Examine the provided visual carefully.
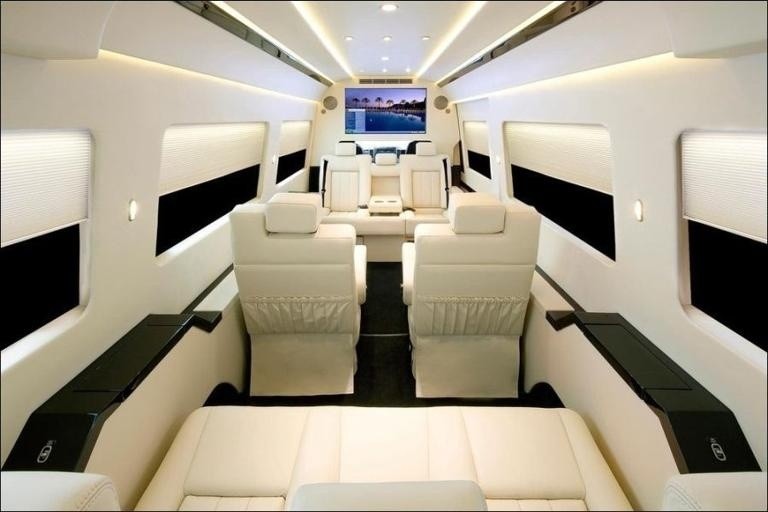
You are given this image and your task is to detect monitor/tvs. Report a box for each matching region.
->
[345,88,427,134]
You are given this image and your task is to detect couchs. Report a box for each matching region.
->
[0,399,765,511]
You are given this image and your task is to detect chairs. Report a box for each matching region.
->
[233,192,368,396]
[402,194,540,399]
[315,142,475,263]
